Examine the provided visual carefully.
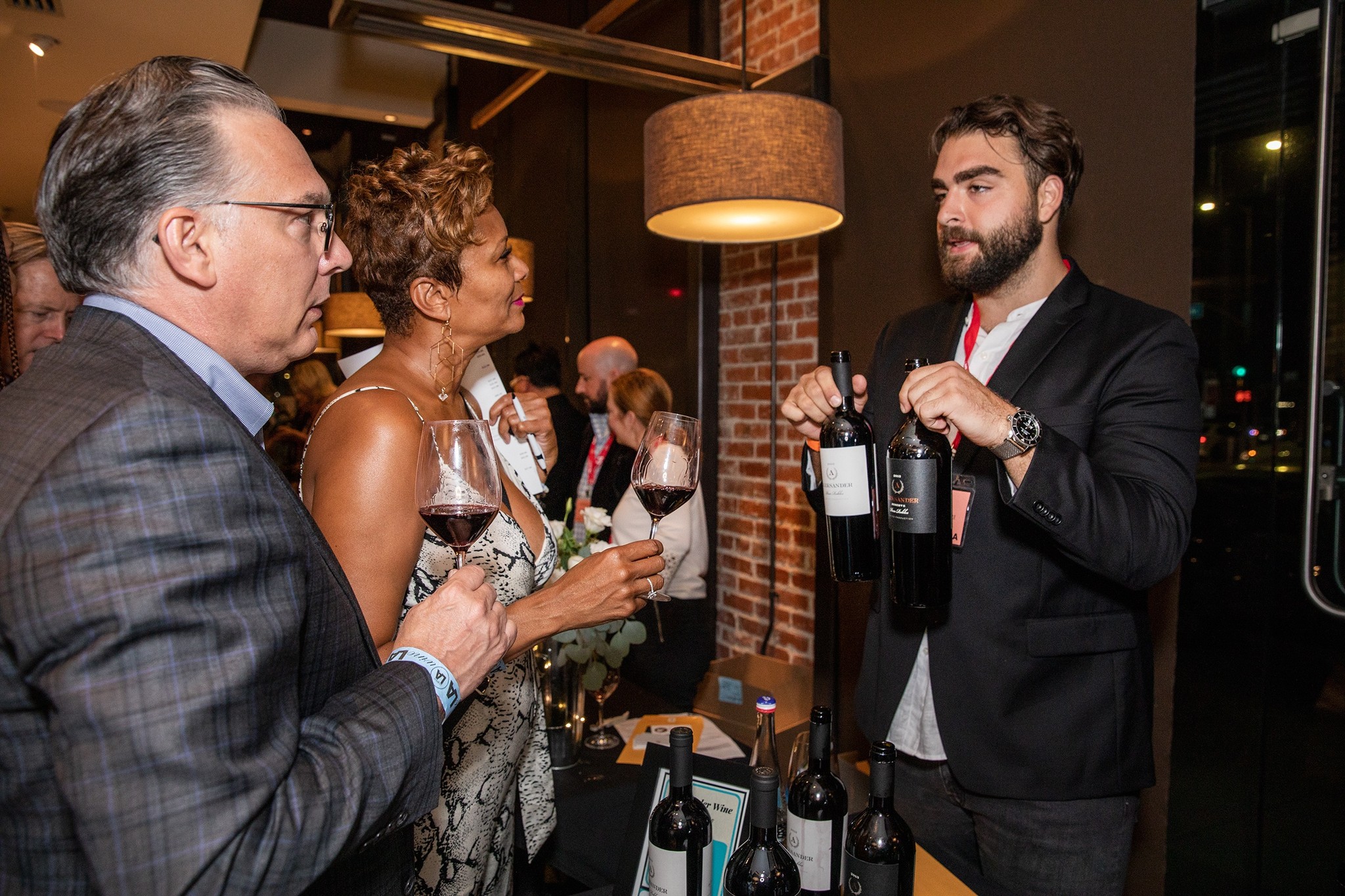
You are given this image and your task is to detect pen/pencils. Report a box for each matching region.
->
[510,392,547,474]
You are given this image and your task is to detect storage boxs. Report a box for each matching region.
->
[691,652,872,814]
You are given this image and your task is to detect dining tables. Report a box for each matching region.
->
[542,671,982,896]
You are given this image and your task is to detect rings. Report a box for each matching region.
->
[647,578,658,600]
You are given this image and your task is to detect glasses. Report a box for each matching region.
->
[153,200,336,252]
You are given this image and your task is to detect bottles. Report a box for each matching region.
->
[818,346,884,584]
[646,688,917,896]
[888,355,953,611]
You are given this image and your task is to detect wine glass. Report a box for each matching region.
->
[582,668,622,750]
[632,411,704,603]
[414,413,503,568]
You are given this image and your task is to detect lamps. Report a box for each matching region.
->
[322,291,387,338]
[311,320,341,354]
[26,33,62,56]
[643,90,846,243]
[504,236,537,303]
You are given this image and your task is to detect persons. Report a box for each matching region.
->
[0,219,77,382]
[1,57,515,896]
[599,367,721,713]
[563,336,640,542]
[779,91,1199,896]
[503,341,593,535]
[295,137,667,893]
[262,358,340,489]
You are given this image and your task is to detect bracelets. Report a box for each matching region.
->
[387,647,461,720]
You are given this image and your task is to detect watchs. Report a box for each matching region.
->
[988,407,1043,460]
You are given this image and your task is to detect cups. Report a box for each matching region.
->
[545,705,582,772]
